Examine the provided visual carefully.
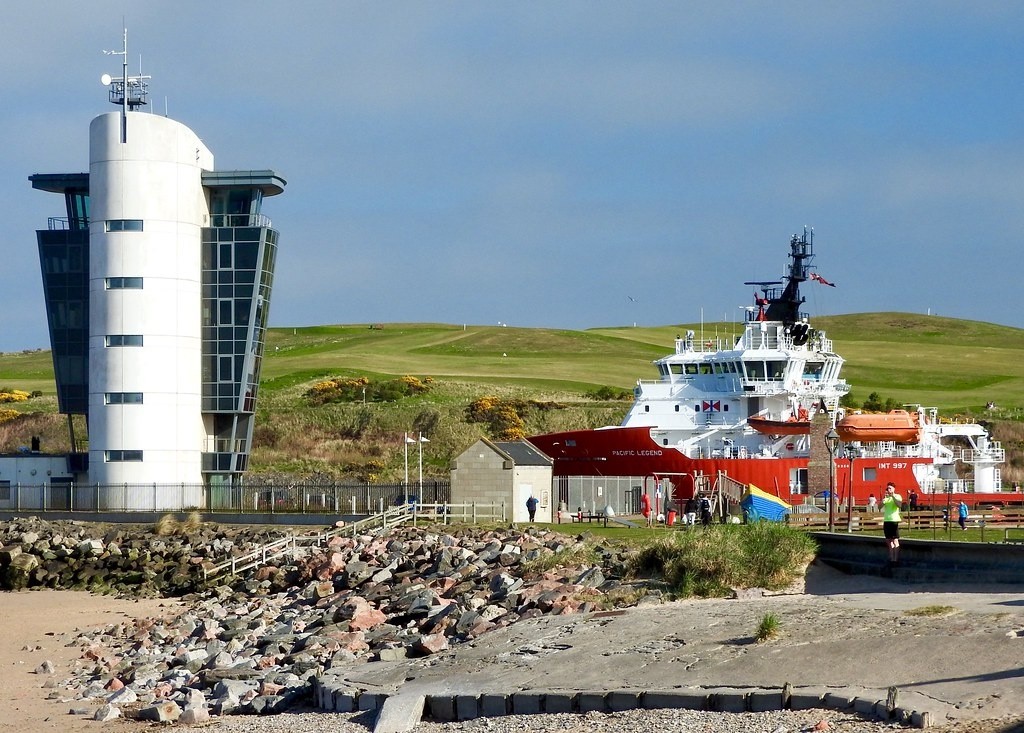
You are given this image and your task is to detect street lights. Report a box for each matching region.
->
[419,437,430,511]
[824,427,840,534]
[844,442,859,533]
[404,437,416,512]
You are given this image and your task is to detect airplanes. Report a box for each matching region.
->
[628,296,639,303]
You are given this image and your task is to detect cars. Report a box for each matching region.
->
[394,494,419,512]
[740,483,793,522]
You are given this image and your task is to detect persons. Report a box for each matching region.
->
[909,489,919,511]
[525,495,539,522]
[951,499,969,530]
[877,481,903,566]
[684,497,699,525]
[699,493,711,525]
[867,494,878,513]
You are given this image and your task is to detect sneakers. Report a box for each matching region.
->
[889,561,899,567]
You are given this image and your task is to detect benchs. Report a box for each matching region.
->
[571,510,609,523]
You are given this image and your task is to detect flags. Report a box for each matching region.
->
[809,272,836,288]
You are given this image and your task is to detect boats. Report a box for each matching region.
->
[526,223,1024,516]
